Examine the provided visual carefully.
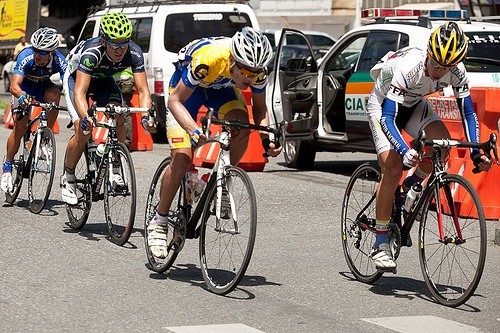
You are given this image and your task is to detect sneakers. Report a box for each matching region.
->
[39,147,52,160]
[1,163,13,193]
[214,185,230,219]
[62,174,78,206]
[368,243,397,269]
[146,216,168,259]
[414,198,425,221]
[113,174,124,192]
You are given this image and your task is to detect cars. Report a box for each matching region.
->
[263,8,500,168]
[0,44,69,92]
[264,29,336,61]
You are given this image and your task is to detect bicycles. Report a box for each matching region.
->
[340,128,499,307]
[6,101,74,214]
[143,107,289,296]
[62,101,159,245]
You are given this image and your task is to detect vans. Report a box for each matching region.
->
[65,0,261,126]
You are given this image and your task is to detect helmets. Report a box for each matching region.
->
[30,26,59,52]
[100,11,133,40]
[230,26,273,70]
[427,22,470,69]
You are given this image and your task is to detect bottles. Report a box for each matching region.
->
[403,181,422,213]
[190,172,211,202]
[185,166,198,205]
[88,140,98,171]
[94,143,104,165]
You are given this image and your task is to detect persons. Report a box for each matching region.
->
[148,25,282,258]
[366,21,491,270]
[13,36,33,60]
[0,26,69,194]
[60,11,156,205]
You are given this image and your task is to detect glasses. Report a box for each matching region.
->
[235,61,264,78]
[428,52,457,71]
[102,36,130,49]
[31,46,49,55]
